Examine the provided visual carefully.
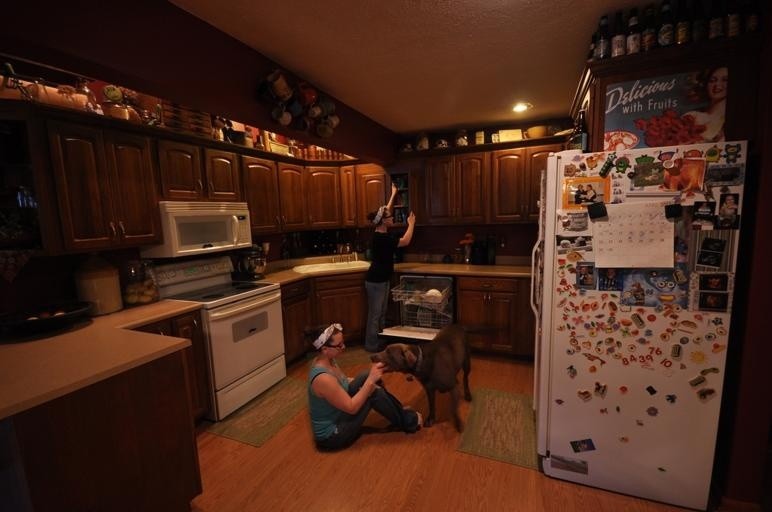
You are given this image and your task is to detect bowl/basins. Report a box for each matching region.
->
[8,299,95,333]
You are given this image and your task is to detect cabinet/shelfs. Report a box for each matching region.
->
[492,137,563,224]
[302,160,342,229]
[241,147,306,235]
[340,160,356,229]
[357,149,426,228]
[157,129,240,202]
[427,143,492,225]
[1,95,164,253]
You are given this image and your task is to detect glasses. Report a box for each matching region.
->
[325,343,343,348]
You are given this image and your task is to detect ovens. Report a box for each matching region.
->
[202,289,287,424]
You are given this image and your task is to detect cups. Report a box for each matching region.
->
[523,125,547,138]
[264,69,342,137]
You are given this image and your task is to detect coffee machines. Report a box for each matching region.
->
[237,242,267,281]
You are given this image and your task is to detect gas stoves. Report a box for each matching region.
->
[163,279,278,309]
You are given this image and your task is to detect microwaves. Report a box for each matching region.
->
[139,199,253,260]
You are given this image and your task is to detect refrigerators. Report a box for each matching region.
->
[530,139,749,510]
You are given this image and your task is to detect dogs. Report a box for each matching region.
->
[369,325,473,433]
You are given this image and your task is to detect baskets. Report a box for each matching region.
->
[391,280,451,304]
[400,309,453,329]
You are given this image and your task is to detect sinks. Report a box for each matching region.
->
[293,259,371,275]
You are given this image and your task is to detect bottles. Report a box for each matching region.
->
[588,0,764,60]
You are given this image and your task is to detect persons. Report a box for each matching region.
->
[584,184,596,201]
[721,195,737,217]
[599,269,618,290]
[306,322,423,453]
[580,267,588,282]
[575,184,585,202]
[683,68,727,141]
[353,181,417,354]
